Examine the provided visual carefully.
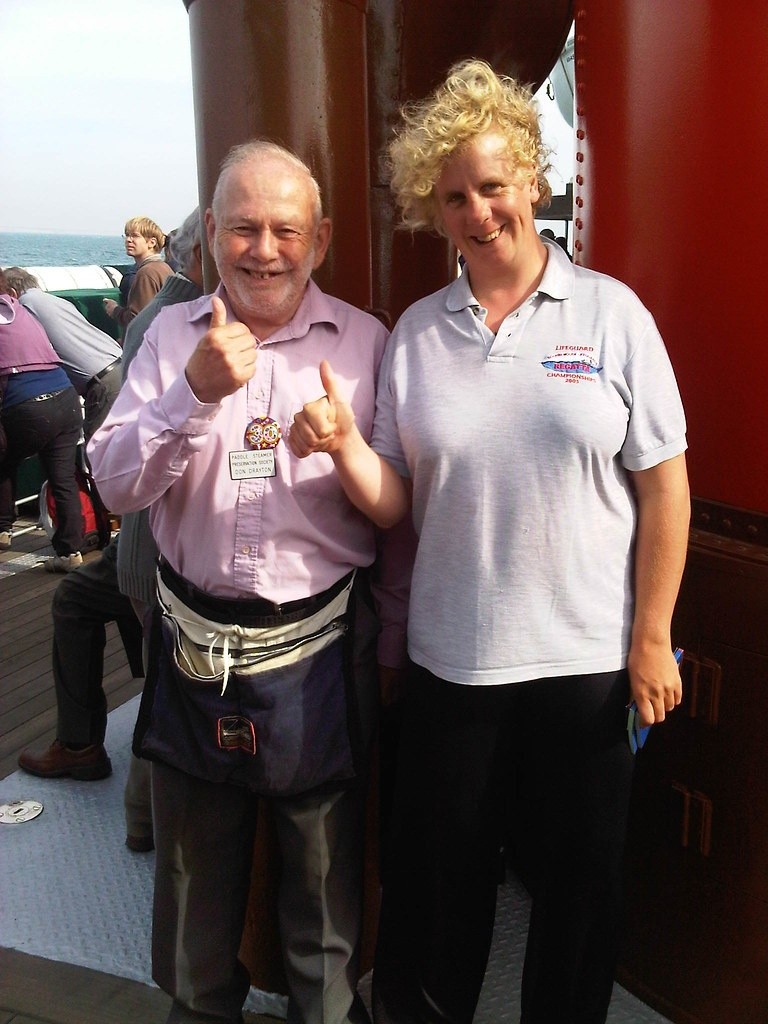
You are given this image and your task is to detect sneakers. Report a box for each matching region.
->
[45,551,84,572]
[0,532,12,550]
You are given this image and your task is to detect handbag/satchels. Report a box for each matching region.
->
[131,569,357,796]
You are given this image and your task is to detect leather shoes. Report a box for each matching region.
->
[18,739,113,781]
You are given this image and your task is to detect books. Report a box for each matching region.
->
[626,649,684,754]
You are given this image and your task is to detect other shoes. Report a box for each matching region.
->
[126,834,154,852]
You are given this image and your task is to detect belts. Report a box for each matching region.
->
[161,558,354,618]
[87,358,122,388]
[33,390,64,401]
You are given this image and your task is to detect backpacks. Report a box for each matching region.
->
[39,462,111,555]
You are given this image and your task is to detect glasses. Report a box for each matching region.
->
[122,233,143,239]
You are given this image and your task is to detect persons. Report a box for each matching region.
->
[291,64,692,1024]
[117,207,205,852]
[0,265,123,575]
[103,216,182,346]
[17,537,126,780]
[86,142,390,1024]
